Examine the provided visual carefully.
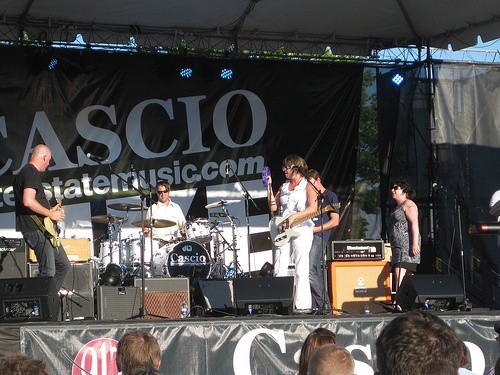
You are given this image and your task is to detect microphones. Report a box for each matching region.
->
[131,164,134,180]
[86,153,107,161]
[291,164,303,170]
[225,160,229,178]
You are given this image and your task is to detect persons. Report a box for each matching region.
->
[299,328,337,375]
[144,180,186,247]
[267,155,317,312]
[13,144,70,293]
[494,322,500,375]
[388,180,421,286]
[115,330,161,375]
[307,170,339,309]
[126,365,160,375]
[308,343,355,375]
[375,310,464,375]
[0,354,48,375]
[489,190,500,245]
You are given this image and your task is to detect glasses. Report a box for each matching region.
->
[282,166,292,171]
[158,190,168,195]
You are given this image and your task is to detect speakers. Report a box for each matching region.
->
[96,285,141,321]
[394,273,464,311]
[190,278,234,317]
[327,260,391,314]
[28,263,94,320]
[0,238,29,278]
[135,277,190,318]
[0,276,61,323]
[231,276,297,314]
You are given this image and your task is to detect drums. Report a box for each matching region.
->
[101,239,129,269]
[152,241,212,279]
[185,217,212,246]
[125,238,160,266]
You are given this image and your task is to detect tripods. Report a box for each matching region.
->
[96,160,172,321]
[301,170,358,315]
[204,206,248,279]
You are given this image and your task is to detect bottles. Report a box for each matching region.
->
[227,268,235,278]
[181,299,187,318]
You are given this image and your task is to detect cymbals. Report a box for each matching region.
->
[130,219,177,228]
[205,199,242,209]
[262,166,271,187]
[107,203,148,211]
[90,216,128,224]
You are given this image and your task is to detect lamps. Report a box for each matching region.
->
[389,66,407,88]
[179,56,194,78]
[219,61,238,82]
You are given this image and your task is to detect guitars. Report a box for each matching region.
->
[44,199,62,247]
[268,201,340,247]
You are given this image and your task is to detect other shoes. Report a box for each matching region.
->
[292,309,313,315]
[383,304,403,312]
